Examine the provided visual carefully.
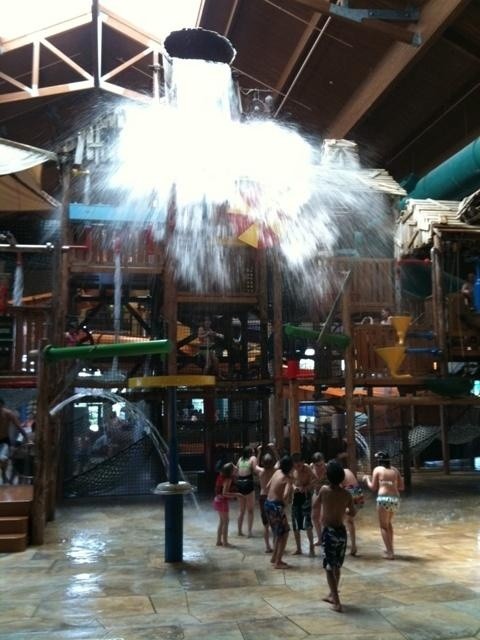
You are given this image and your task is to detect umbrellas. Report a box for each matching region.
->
[227,187,285,251]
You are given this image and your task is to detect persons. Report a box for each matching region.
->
[213,441,366,570]
[198,316,228,382]
[460,272,476,312]
[361,451,405,561]
[380,306,395,325]
[0,397,36,485]
[91,411,123,455]
[308,458,358,614]
